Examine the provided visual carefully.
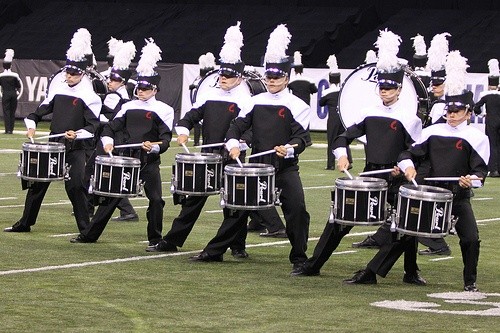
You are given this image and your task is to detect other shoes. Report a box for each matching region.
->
[4,226,12,232]
[247,224,267,232]
[326,166,335,170]
[341,164,353,173]
[259,228,287,238]
[489,170,499,177]
[111,214,139,222]
[149,241,158,246]
[70,236,84,243]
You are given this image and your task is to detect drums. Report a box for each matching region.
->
[221,163,282,210]
[390,185,459,238]
[192,70,267,125]
[329,177,392,225]
[88,155,146,198]
[17,142,72,182]
[170,153,222,196]
[337,62,428,144]
[46,66,108,98]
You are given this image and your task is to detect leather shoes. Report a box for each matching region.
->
[403,273,427,285]
[189,250,224,263]
[232,249,249,258]
[351,236,379,249]
[343,270,378,286]
[292,260,306,270]
[145,240,178,252]
[419,245,451,256]
[464,283,478,293]
[289,261,320,277]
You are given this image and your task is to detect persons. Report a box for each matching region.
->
[247,208,287,238]
[189,67,214,146]
[71,73,174,246]
[290,70,427,286]
[287,64,317,145]
[4,59,103,230]
[0,61,23,133]
[343,91,490,292]
[189,60,311,270]
[474,74,500,177]
[85,54,137,103]
[319,72,353,171]
[145,62,249,257]
[71,69,140,221]
[353,57,451,255]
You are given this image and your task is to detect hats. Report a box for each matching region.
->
[65,58,88,74]
[443,89,474,111]
[488,75,499,86]
[290,63,304,74]
[218,61,245,76]
[328,72,341,84]
[109,68,132,83]
[430,67,446,85]
[378,69,404,89]
[265,60,291,79]
[200,67,214,76]
[2,62,12,69]
[412,53,428,67]
[135,74,162,91]
[6,130,12,134]
